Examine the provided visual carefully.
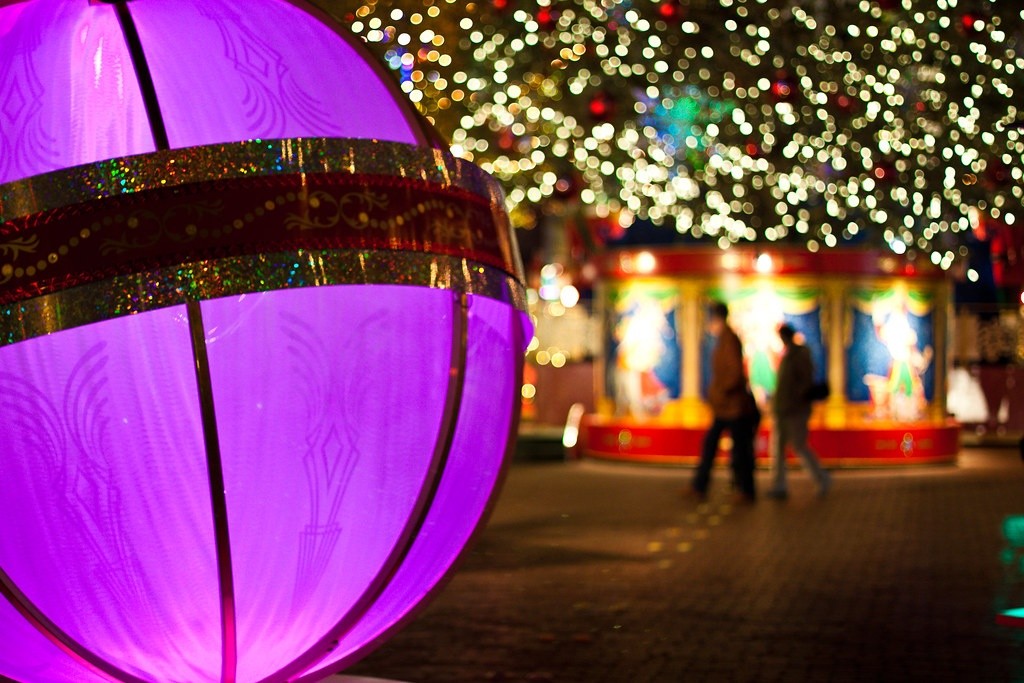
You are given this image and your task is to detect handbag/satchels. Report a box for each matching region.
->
[809,379,830,401]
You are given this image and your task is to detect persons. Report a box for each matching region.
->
[766,322,832,500]
[680,302,756,503]
